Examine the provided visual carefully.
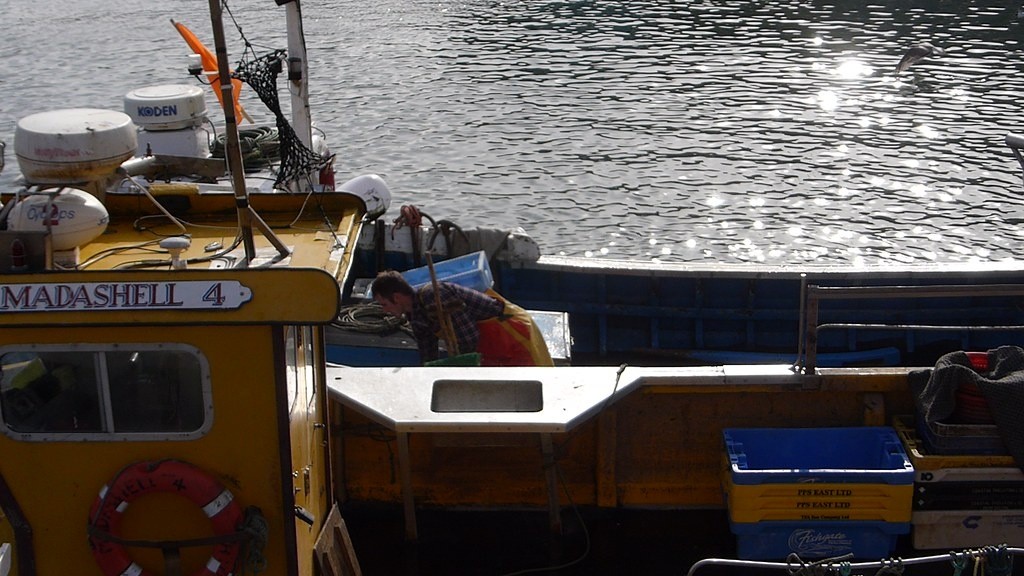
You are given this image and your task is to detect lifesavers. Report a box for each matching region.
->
[90,461,242,576]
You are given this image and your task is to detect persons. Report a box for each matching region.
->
[372,271,555,367]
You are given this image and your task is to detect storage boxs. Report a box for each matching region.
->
[715,408,1024,563]
[404,250,495,292]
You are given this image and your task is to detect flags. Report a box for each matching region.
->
[173,22,243,125]
